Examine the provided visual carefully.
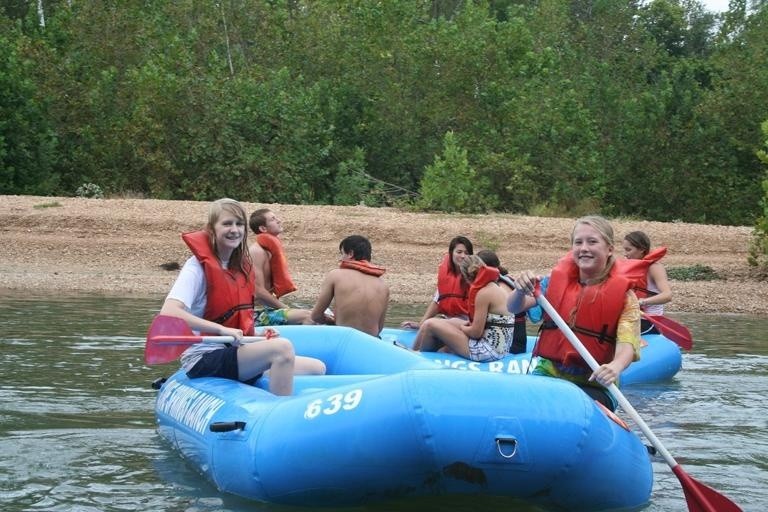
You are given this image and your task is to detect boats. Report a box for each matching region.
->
[379,324,681,385]
[154,325,654,512]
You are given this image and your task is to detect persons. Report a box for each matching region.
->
[400,236,473,351]
[310,234,389,338]
[247,209,319,328]
[160,197,327,395]
[622,231,673,336]
[414,250,516,363]
[506,215,642,414]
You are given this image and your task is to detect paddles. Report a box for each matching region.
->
[144,316,274,366]
[532,278,742,512]
[640,310,693,350]
